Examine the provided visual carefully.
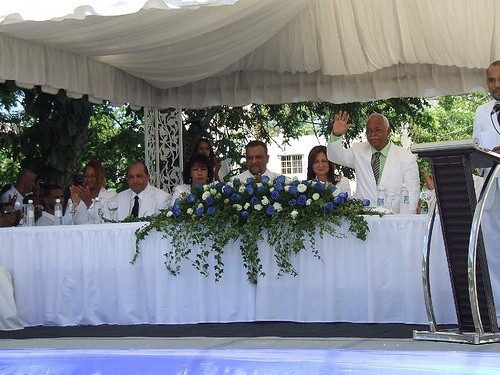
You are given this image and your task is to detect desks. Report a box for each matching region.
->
[0,215,457,330]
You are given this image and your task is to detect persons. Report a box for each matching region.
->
[0,139,221,227]
[327,111,420,213]
[471,60,500,178]
[229,140,282,184]
[420,167,483,214]
[307,145,351,197]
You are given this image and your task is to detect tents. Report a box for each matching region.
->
[0,0,500,196]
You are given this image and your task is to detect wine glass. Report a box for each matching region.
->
[107,201,118,223]
[66,203,78,225]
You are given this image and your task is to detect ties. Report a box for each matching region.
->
[372,152,381,182]
[129,196,139,218]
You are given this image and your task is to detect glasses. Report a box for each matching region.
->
[3,210,15,215]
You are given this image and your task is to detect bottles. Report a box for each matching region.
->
[54,199,63,225]
[377,186,386,207]
[94,197,102,224]
[26,199,35,226]
[399,184,410,214]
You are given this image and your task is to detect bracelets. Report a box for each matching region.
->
[333,132,343,137]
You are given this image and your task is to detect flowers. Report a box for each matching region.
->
[98,175,393,284]
